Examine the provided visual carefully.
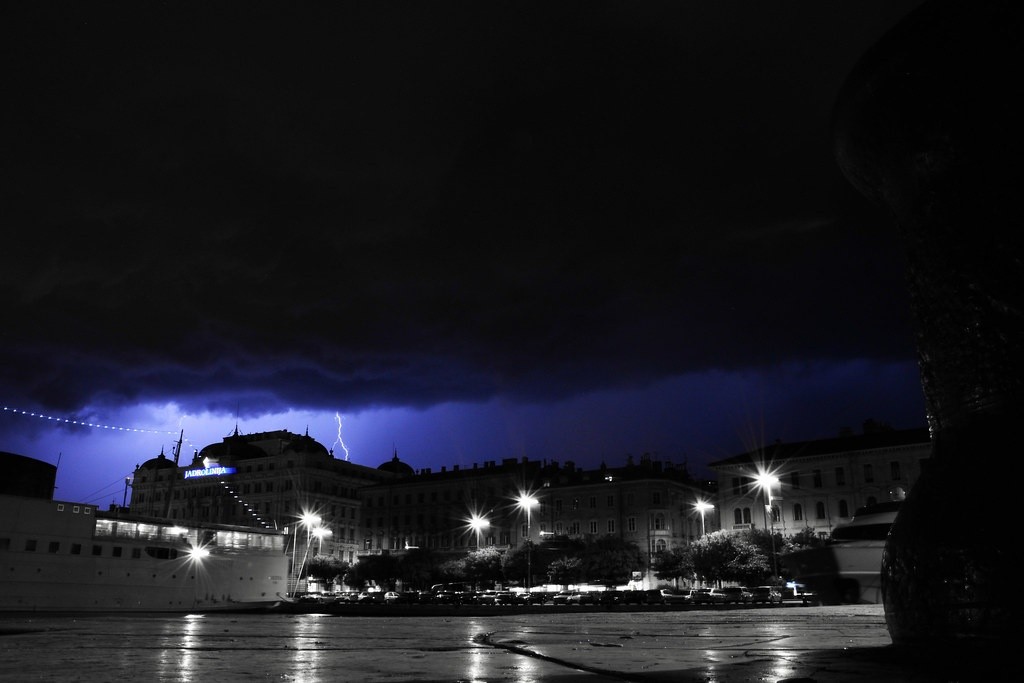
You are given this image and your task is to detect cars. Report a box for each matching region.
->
[286,592,309,602]
[320,591,339,604]
[478,586,823,607]
[350,593,358,602]
[335,591,356,604]
[301,591,322,602]
[384,591,409,604]
[358,591,375,602]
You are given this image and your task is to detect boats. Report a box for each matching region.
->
[0,492,291,618]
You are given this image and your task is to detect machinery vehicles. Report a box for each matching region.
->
[431,581,477,605]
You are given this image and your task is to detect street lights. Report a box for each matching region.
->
[123,477,130,507]
[695,501,707,538]
[316,528,326,558]
[759,472,781,586]
[521,494,535,589]
[471,516,485,551]
[301,512,315,558]
